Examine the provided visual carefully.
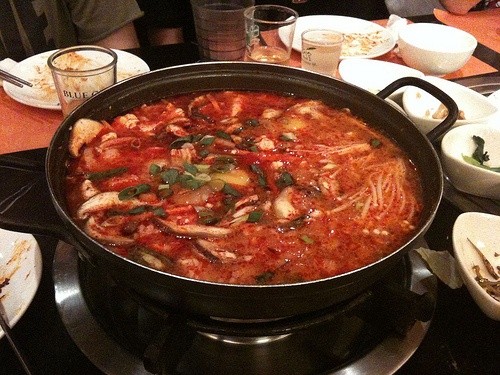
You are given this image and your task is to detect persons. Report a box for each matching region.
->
[0,0,499,66]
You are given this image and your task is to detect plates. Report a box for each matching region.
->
[0,229,42,337]
[2,49,150,111]
[278,14,396,60]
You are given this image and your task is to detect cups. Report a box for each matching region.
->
[48,44,118,120]
[301,30,344,78]
[244,5,298,66]
[190,0,255,62]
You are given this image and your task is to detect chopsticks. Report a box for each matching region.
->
[0,69,33,87]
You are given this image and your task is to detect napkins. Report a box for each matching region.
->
[0,86,64,155]
[433,7,500,54]
[260,18,498,80]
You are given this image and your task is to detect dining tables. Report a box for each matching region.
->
[0,13,500,375]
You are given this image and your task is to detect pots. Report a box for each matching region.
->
[43,61,458,317]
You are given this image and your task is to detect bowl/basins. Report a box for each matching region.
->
[453,212,500,323]
[399,22,477,75]
[401,77,498,139]
[440,124,500,200]
[339,59,425,100]
